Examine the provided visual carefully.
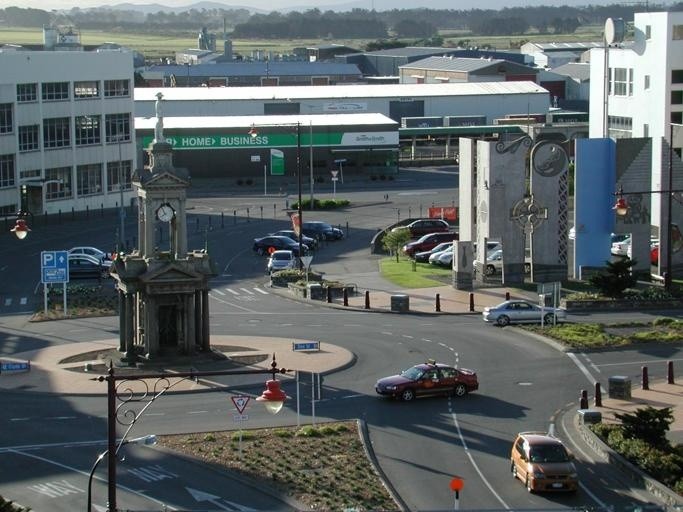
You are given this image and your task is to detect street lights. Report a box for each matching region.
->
[87,434,157,512]
[0,210,34,241]
[611,184,683,216]
[87,352,297,512]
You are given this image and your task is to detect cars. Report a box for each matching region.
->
[252,221,344,276]
[511,431,579,494]
[568,226,659,267]
[482,300,567,327]
[374,358,479,401]
[67,246,114,279]
[391,218,531,277]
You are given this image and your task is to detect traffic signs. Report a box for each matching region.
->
[293,340,321,353]
[41,250,69,284]
[0,362,31,373]
